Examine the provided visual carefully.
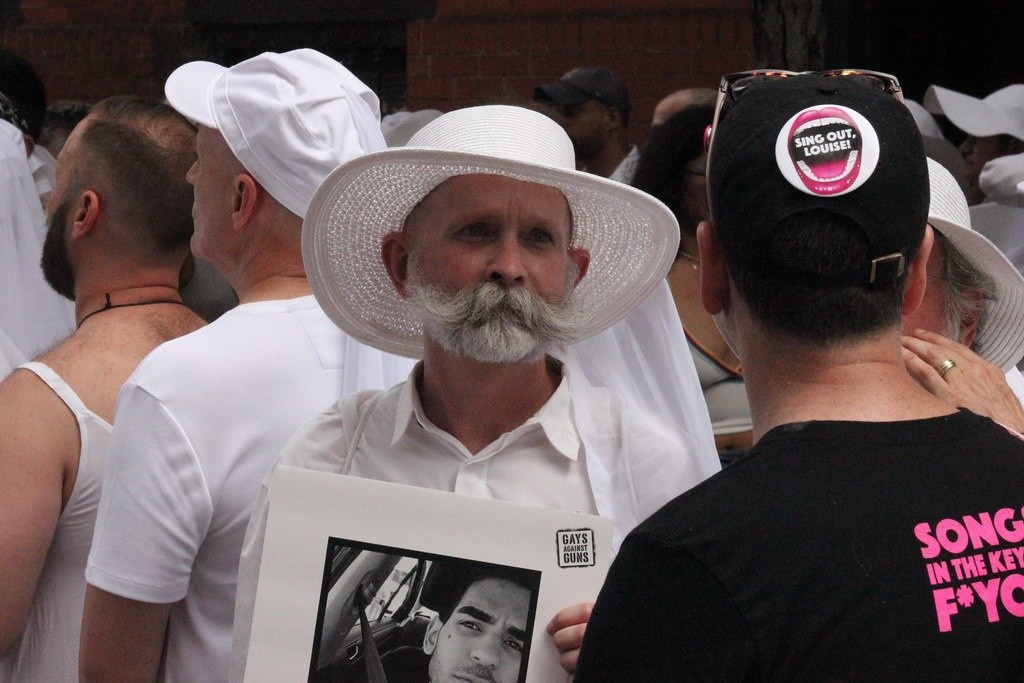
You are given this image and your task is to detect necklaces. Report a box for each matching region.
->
[77,293,185,330]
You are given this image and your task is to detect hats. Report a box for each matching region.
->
[711,74,929,284]
[927,156,1024,375]
[978,152,1024,208]
[301,104,681,358]
[903,99,969,193]
[380,110,443,148]
[533,65,632,114]
[923,84,1024,142]
[163,47,388,219]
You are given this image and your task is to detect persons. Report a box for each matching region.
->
[534,65,640,186]
[0,49,95,378]
[0,94,210,683]
[78,48,420,683]
[571,67,1024,683]
[227,105,683,683]
[423,562,532,683]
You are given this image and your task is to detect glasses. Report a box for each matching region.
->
[546,103,605,118]
[705,68,905,246]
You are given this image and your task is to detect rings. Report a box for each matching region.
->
[938,359,955,378]
